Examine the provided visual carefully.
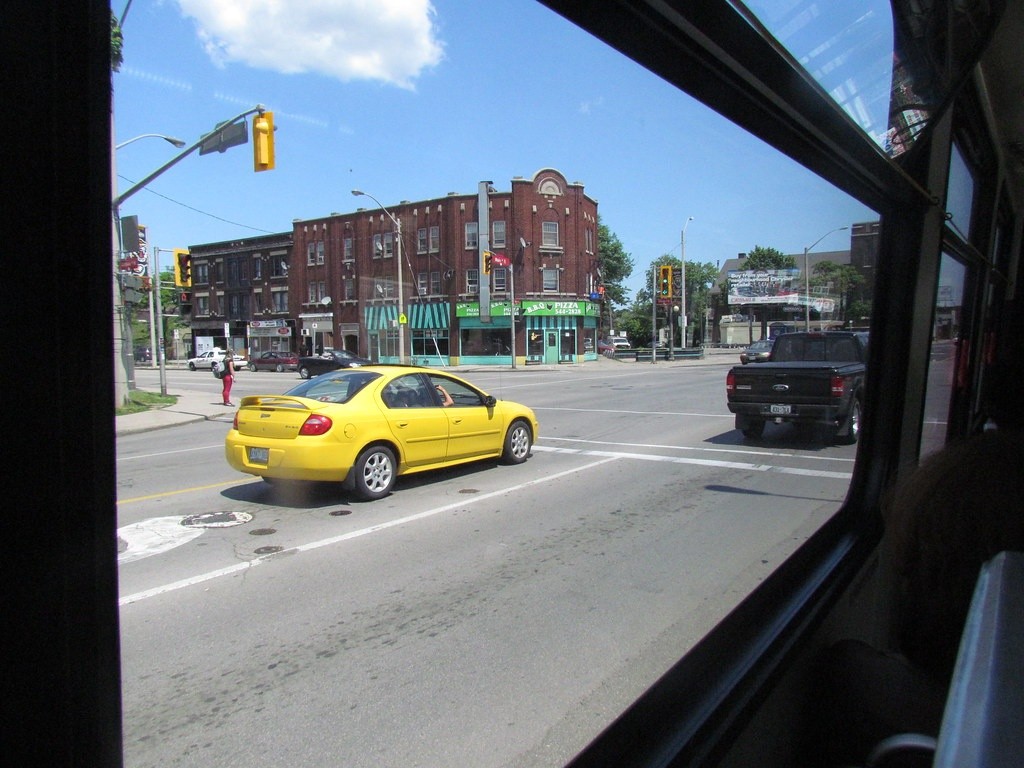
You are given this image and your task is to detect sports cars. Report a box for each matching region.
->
[299,348,374,379]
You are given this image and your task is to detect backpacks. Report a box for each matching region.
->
[213,358,232,379]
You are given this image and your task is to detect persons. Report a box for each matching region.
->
[416,380,454,408]
[221,349,237,406]
[299,341,309,357]
[878,424,1024,670]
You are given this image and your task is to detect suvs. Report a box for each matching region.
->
[610,337,631,349]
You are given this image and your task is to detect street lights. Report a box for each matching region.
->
[115,133,186,151]
[351,190,405,366]
[483,251,492,274]
[681,216,694,349]
[804,226,849,331]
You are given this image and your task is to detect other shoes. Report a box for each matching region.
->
[226,402,234,407]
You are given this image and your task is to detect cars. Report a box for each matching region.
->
[598,340,615,354]
[187,347,248,373]
[740,340,775,366]
[225,364,540,500]
[247,351,299,373]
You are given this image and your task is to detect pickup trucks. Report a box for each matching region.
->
[724,331,869,446]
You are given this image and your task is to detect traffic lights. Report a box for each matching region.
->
[174,249,193,287]
[179,292,193,317]
[660,266,672,299]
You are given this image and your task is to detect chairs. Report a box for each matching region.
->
[386,386,422,407]
